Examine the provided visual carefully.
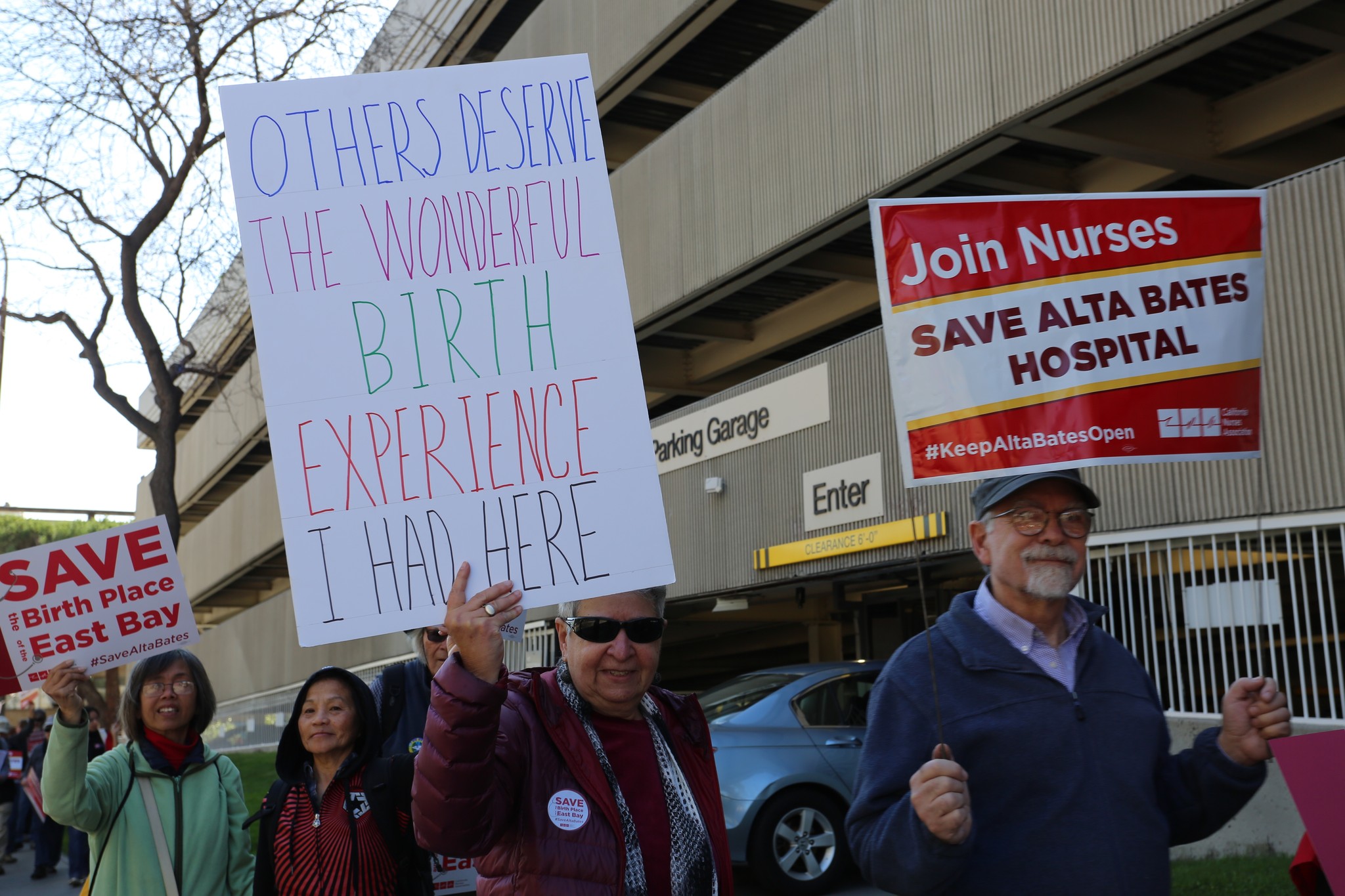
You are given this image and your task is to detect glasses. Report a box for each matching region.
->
[423,626,448,643]
[141,680,195,698]
[978,505,1097,539]
[562,616,667,643]
[45,727,52,732]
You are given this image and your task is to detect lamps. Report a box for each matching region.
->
[704,477,723,494]
[795,587,805,608]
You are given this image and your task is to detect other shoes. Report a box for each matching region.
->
[0,866,6,876]
[31,868,58,880]
[4,854,16,864]
[68,876,86,887]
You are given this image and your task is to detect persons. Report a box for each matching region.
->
[845,470,1294,896]
[0,560,733,896]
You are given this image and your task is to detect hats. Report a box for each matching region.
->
[970,467,1102,522]
[43,715,55,729]
[0,717,10,733]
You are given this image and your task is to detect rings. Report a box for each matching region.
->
[482,604,495,617]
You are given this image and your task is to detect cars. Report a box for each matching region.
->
[694,656,887,896]
